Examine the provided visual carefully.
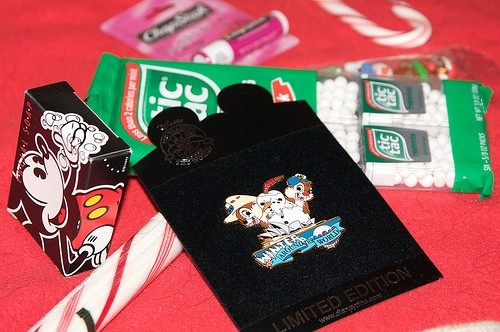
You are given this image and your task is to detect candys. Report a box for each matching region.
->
[316,0,453,189]
